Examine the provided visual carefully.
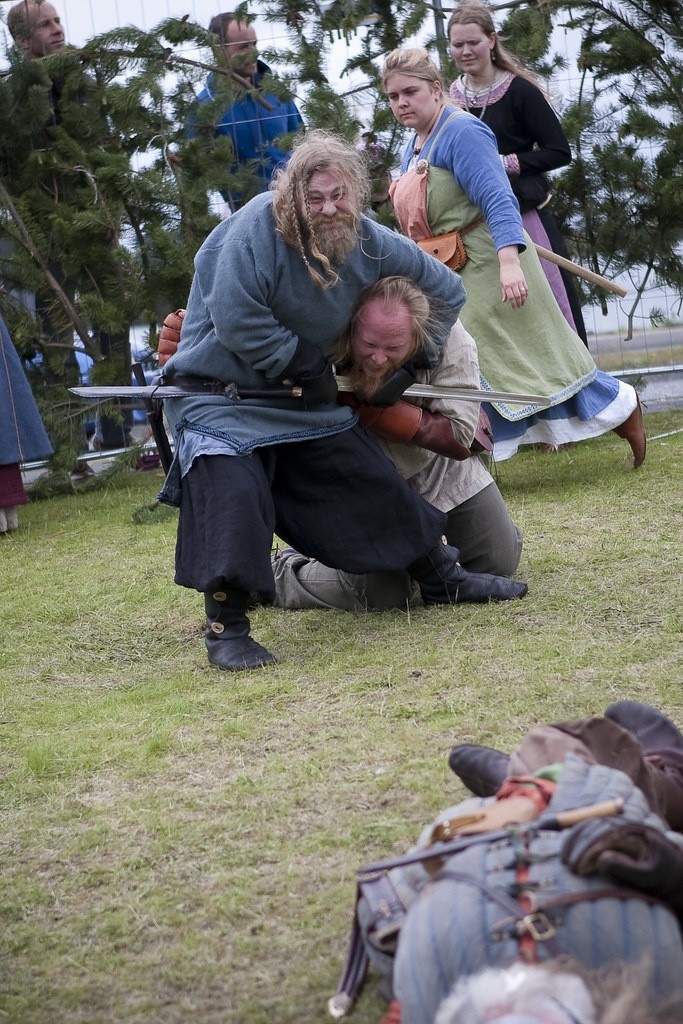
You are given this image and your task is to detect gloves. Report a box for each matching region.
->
[561,814,683,914]
[157,307,190,367]
[301,360,338,409]
[365,366,415,407]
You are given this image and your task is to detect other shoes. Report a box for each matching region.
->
[605,701,683,778]
[90,434,160,470]
[0,506,19,536]
[611,392,646,470]
[449,744,511,798]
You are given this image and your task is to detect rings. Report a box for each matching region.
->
[520,288,525,291]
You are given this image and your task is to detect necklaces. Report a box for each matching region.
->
[465,72,495,121]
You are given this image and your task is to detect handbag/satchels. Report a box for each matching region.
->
[418,230,467,272]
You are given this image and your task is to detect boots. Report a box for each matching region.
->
[407,533,528,607]
[204,577,277,672]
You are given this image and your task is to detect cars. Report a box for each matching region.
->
[25,326,165,434]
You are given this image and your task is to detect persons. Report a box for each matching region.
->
[328,702,683,1023]
[381,48,646,468]
[445,3,588,355]
[179,13,307,215]
[7,0,159,482]
[358,133,394,186]
[158,278,522,612]
[156,134,530,669]
[0,319,54,533]
